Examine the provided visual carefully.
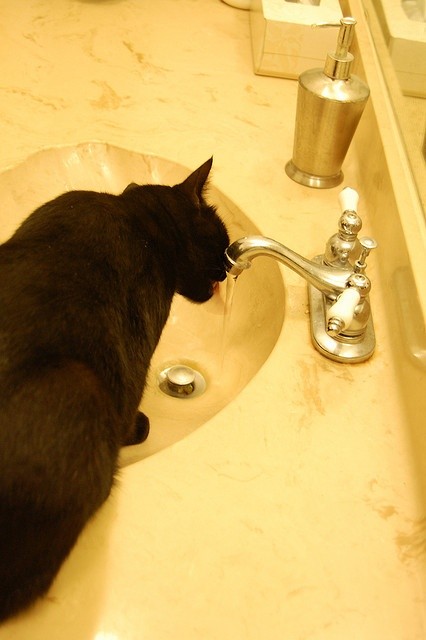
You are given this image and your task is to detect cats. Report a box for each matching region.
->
[1,154,230,623]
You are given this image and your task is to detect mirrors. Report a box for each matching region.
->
[357,0,426,220]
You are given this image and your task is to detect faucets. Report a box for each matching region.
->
[224,202,376,365]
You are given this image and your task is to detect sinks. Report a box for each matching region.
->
[0,136,288,483]
